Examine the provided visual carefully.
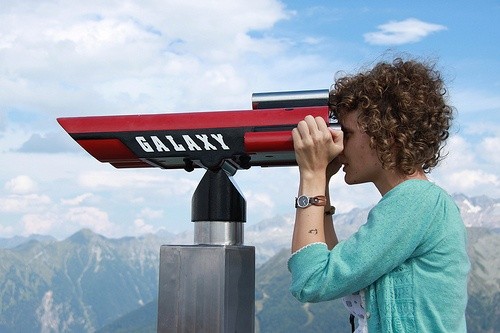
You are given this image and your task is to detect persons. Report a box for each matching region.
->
[287,59,472,333]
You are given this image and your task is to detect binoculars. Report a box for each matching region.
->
[56,89,345,172]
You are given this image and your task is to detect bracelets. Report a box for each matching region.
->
[325,206,335,215]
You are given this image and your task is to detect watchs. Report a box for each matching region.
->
[294,195,328,209]
